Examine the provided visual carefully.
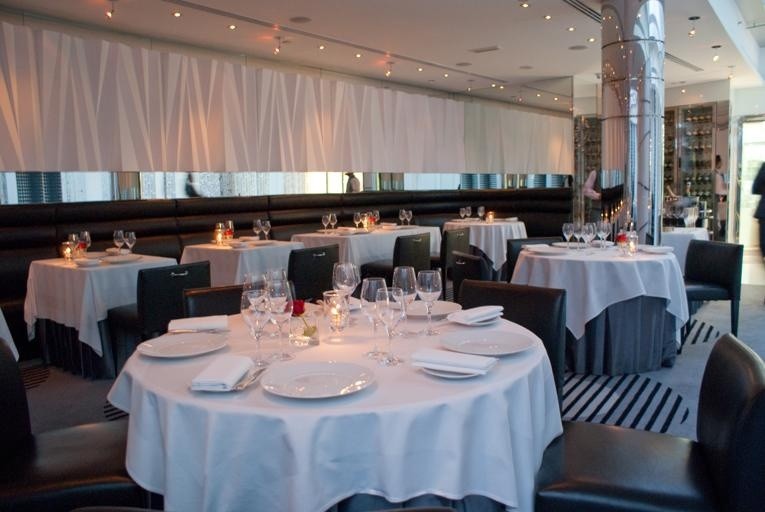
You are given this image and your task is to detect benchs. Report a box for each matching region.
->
[0,187,579,383]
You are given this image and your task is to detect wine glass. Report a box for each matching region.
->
[60,228,136,263]
[563,219,638,256]
[316,208,413,235]
[458,204,495,224]
[237,259,444,371]
[213,218,272,249]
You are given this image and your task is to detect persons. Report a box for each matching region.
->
[583,169,603,222]
[752,161,765,260]
[714,155,727,202]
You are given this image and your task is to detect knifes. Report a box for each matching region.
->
[234,366,266,391]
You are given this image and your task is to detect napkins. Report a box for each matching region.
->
[402,347,497,376]
[189,353,256,393]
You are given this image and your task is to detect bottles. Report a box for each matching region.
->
[575,113,713,233]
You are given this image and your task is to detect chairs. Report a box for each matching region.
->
[677,240,744,345]
[535,332,765,511]
[0,338,163,510]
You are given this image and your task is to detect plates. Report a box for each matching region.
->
[259,357,377,399]
[403,300,462,320]
[447,311,501,326]
[436,328,534,357]
[133,331,227,359]
[421,365,477,380]
[525,240,674,256]
[70,255,141,267]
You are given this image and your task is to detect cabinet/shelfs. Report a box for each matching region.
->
[574,104,721,234]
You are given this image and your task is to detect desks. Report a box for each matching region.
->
[107,301,565,511]
[510,245,688,376]
